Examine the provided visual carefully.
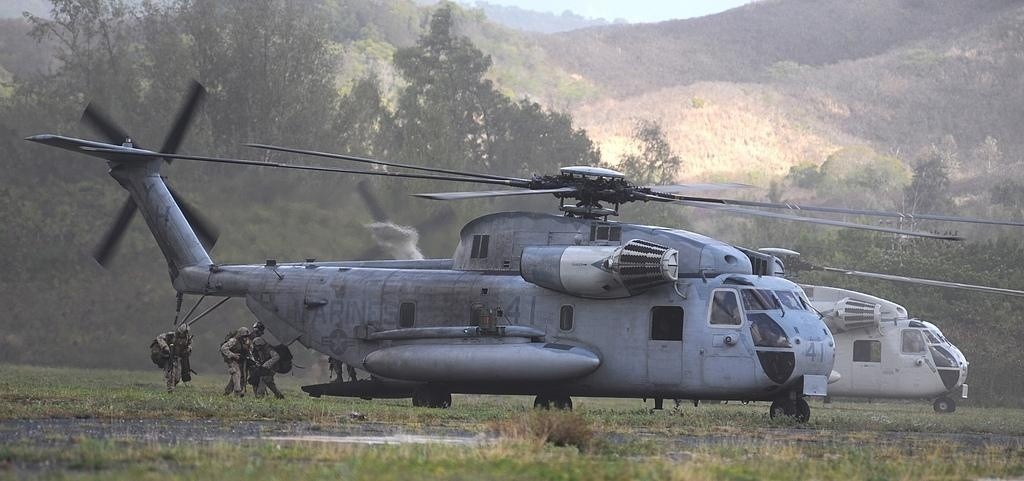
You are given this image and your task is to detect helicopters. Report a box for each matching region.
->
[26,80,1024,427]
[356,173,1024,415]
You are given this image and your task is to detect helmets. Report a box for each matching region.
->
[253,321,264,331]
[238,327,250,337]
[252,336,267,346]
[177,322,190,333]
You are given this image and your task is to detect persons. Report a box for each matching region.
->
[156,323,193,394]
[219,321,284,401]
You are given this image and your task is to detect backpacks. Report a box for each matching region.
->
[269,343,293,375]
[149,338,171,368]
